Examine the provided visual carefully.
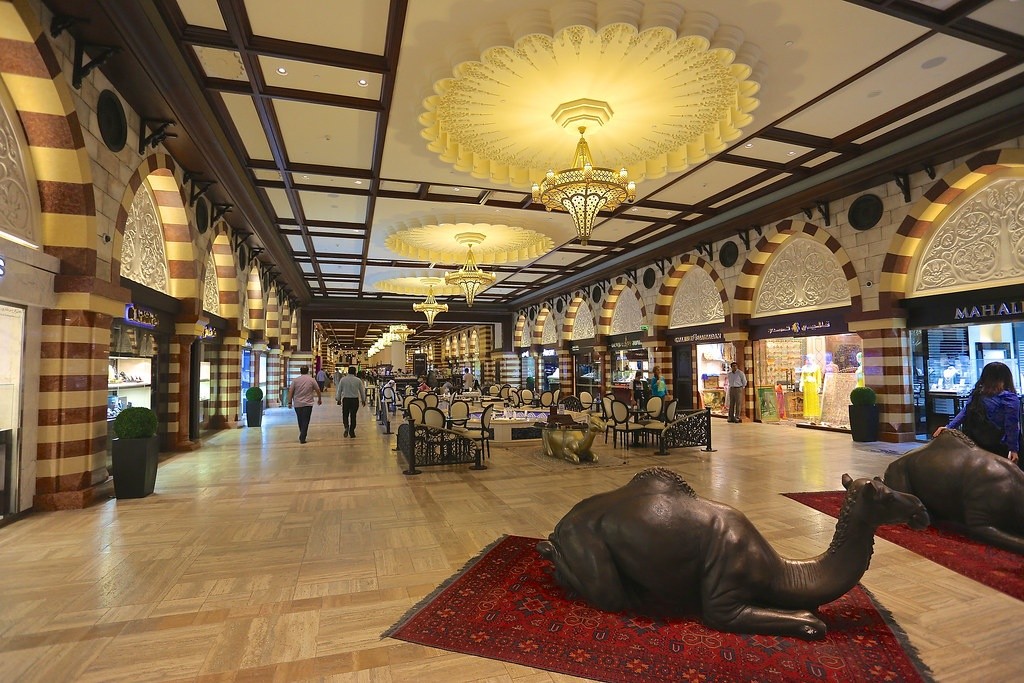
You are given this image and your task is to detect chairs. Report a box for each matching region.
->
[364,379,678,460]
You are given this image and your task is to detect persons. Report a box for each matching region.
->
[855,352,863,387]
[651,366,668,414]
[798,354,821,421]
[932,361,1020,464]
[728,362,747,423]
[428,364,438,387]
[629,371,643,411]
[397,368,404,373]
[317,368,327,392]
[943,366,957,380]
[441,378,453,394]
[821,353,839,374]
[333,368,341,386]
[288,365,322,443]
[335,367,366,437]
[368,369,380,383]
[463,368,481,391]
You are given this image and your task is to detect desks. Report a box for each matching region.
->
[703,389,725,410]
[628,410,656,447]
[524,398,541,408]
[445,418,471,463]
[583,402,601,412]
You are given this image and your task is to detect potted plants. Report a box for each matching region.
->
[246,387,265,426]
[111,407,160,499]
[849,388,877,442]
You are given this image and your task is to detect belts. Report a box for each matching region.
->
[731,387,741,388]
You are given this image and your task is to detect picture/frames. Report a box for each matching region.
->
[755,385,781,422]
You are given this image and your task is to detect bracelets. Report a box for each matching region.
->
[288,403,292,404]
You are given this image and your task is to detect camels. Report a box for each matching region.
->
[540,414,608,464]
[885,428,1024,555]
[534,466,932,644]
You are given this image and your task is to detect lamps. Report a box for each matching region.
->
[413,285,448,329]
[368,322,416,358]
[531,125,636,245]
[444,244,496,308]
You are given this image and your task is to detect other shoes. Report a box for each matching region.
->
[349,430,356,438]
[344,428,349,438]
[735,420,740,423]
[728,420,734,423]
[299,436,307,444]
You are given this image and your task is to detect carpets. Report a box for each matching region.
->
[379,532,939,683]
[779,489,1024,603]
